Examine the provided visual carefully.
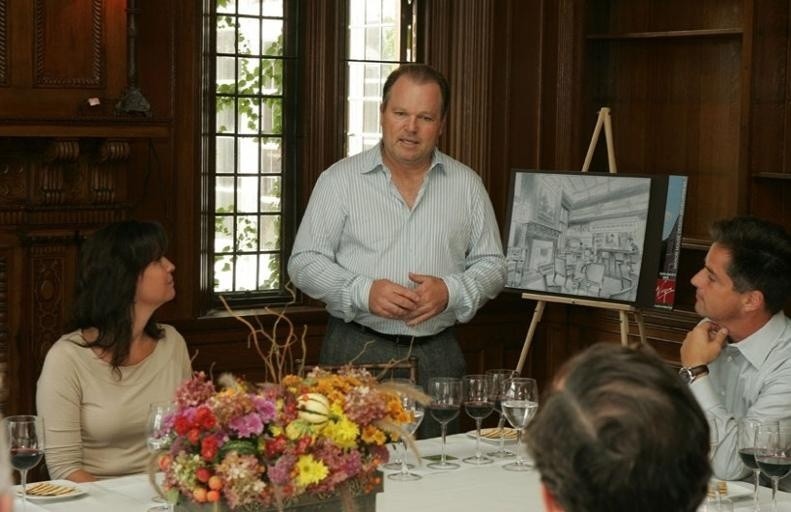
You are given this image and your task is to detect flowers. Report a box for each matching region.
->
[147,361,423,512]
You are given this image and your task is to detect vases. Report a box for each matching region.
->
[173,469,383,512]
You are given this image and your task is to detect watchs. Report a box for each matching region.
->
[678,363,711,384]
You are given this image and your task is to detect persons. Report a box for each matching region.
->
[676,215,790,481]
[36,220,193,484]
[523,337,713,512]
[290,65,503,438]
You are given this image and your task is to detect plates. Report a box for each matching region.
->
[12,480,92,503]
[700,476,753,504]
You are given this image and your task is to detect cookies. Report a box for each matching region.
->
[16,483,78,497]
[706,480,729,496]
[480,427,519,438]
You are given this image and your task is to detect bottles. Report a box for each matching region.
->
[701,481,735,512]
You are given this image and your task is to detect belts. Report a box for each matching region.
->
[349,319,436,346]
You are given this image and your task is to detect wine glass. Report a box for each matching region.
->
[383,370,538,485]
[2,416,48,508]
[736,417,791,510]
